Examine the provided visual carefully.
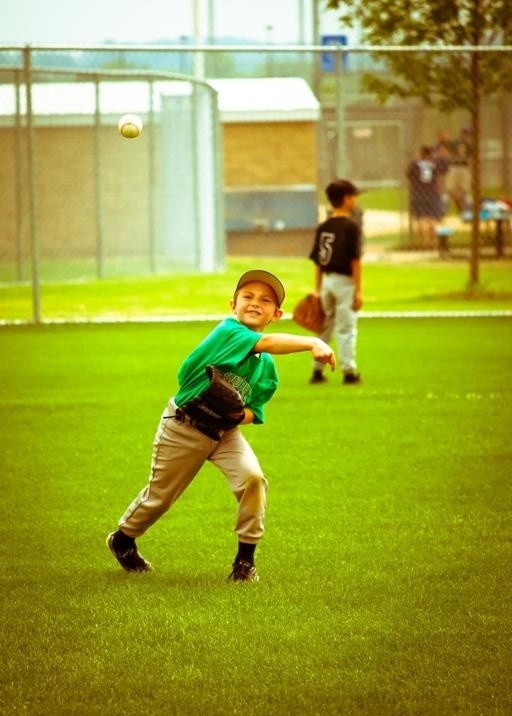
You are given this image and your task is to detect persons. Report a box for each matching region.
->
[104,271,338,580]
[310,180,365,385]
[404,123,474,250]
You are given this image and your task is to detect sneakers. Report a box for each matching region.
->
[106,531,154,573]
[233,559,257,582]
[310,372,360,383]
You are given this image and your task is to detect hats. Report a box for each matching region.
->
[235,269,285,309]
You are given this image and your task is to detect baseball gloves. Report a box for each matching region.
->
[174,365,245,439]
[292,293,324,331]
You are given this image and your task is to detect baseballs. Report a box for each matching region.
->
[118,115,143,138]
